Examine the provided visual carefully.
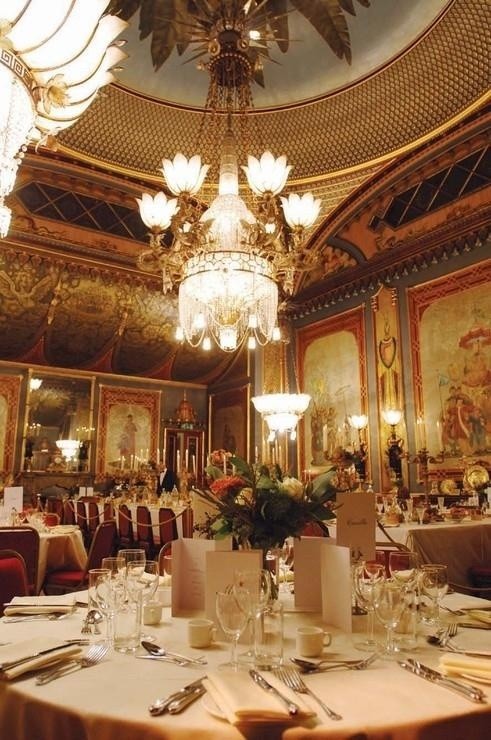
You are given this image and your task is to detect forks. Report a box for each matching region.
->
[139,655,216,669]
[282,668,341,721]
[441,622,458,646]
[34,642,110,686]
[442,605,491,615]
[356,652,386,671]
[80,620,93,637]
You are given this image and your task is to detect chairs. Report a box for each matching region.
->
[0,484,490,605]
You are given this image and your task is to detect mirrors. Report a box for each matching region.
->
[16,368,95,477]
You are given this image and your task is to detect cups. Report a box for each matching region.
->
[112,588,142,655]
[297,627,331,654]
[186,618,214,646]
[143,603,163,627]
[254,607,286,668]
[377,495,487,527]
[11,509,59,533]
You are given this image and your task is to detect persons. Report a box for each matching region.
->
[439,386,485,459]
[156,459,180,497]
[123,414,138,456]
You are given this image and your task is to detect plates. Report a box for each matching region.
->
[457,672,490,690]
[201,691,228,720]
[31,656,64,671]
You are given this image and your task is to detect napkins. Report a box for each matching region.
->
[2,636,81,679]
[436,652,490,682]
[3,596,77,615]
[203,669,317,724]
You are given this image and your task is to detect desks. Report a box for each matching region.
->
[1,575,490,739]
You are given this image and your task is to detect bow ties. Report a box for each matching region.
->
[160,471,164,472]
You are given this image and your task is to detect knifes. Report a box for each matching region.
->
[0,642,79,673]
[247,667,300,716]
[397,657,484,703]
[3,603,69,607]
[149,676,210,716]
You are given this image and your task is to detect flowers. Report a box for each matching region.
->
[189,442,363,552]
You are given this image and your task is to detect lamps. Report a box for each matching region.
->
[132,0,369,353]
[54,380,87,463]
[28,376,45,393]
[250,319,314,450]
[1,0,128,240]
[348,413,372,458]
[378,407,410,488]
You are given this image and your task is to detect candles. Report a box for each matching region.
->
[399,415,447,454]
[318,419,361,455]
[115,444,290,474]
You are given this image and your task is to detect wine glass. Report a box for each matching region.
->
[116,550,148,580]
[124,560,160,602]
[419,564,449,627]
[101,557,127,614]
[216,590,251,671]
[389,552,418,594]
[352,563,388,654]
[371,581,409,661]
[235,569,271,663]
[87,569,112,617]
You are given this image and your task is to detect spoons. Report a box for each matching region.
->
[290,654,356,666]
[4,612,70,624]
[141,639,190,657]
[424,634,491,657]
